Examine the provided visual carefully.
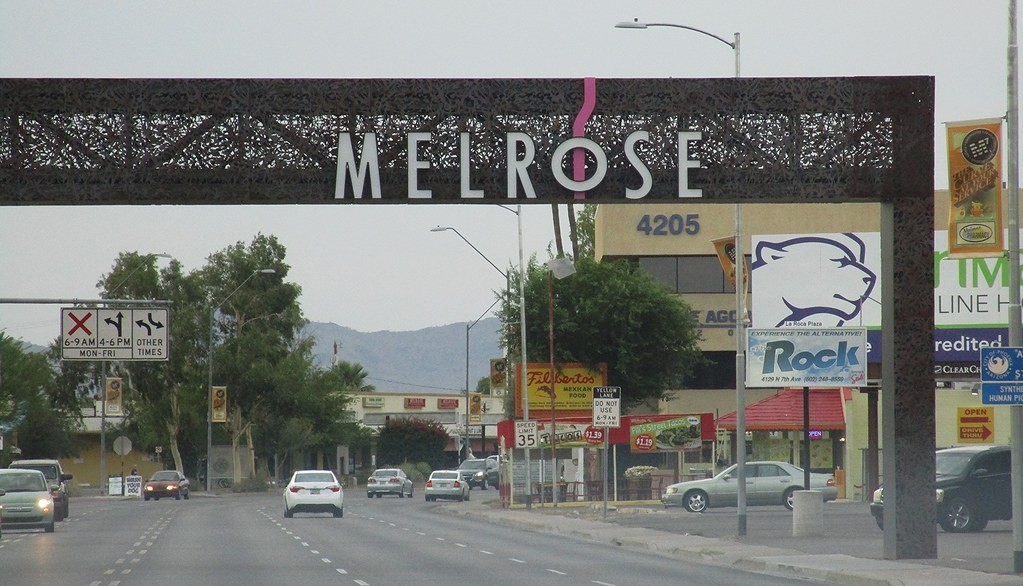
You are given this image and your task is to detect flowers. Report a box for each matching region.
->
[624,465,658,480]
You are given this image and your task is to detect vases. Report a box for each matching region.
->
[627,480,652,501]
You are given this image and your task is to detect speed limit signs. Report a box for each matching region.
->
[514,421,537,449]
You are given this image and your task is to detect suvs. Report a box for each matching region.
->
[456,459,499,491]
[8,460,74,522]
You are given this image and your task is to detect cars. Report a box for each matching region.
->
[486,456,499,462]
[0,469,59,533]
[424,471,471,502]
[142,470,189,500]
[869,443,1011,536]
[284,469,346,518]
[366,468,416,499]
[660,459,838,513]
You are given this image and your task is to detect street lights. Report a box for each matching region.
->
[207,269,275,493]
[100,251,172,497]
[466,288,513,456]
[614,20,748,541]
[431,225,531,510]
